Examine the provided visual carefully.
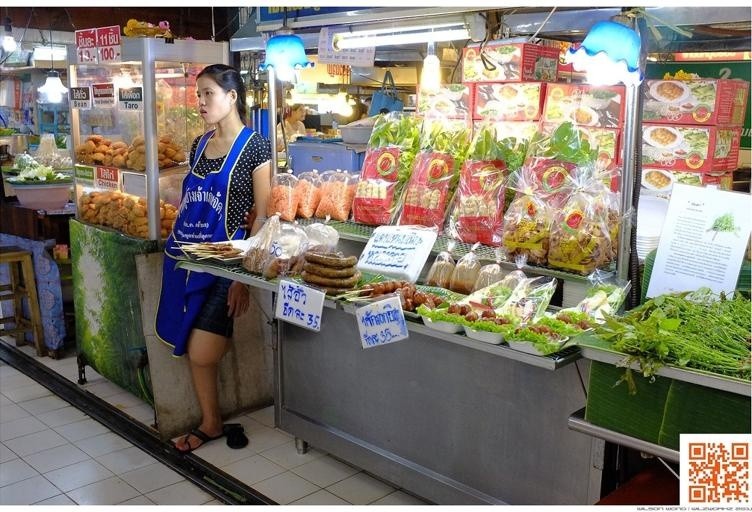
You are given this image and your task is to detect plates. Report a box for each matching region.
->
[564,104,599,126]
[430,97,455,116]
[493,85,524,102]
[476,60,505,81]
[643,126,683,151]
[635,196,671,261]
[640,169,675,193]
[650,80,690,103]
[183,239,252,265]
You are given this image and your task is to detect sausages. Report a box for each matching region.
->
[300,251,360,295]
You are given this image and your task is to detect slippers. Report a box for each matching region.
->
[223,421,249,449]
[175,425,223,453]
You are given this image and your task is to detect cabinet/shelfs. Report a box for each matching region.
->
[0,79,751,506]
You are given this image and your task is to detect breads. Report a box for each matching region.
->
[74,135,186,239]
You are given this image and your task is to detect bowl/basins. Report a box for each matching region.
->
[446,88,464,101]
[10,181,73,210]
[496,46,521,64]
[582,94,611,110]
[420,303,600,357]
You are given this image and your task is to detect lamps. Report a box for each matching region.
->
[0,6,69,104]
[257,7,644,90]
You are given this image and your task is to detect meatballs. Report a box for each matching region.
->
[362,280,590,340]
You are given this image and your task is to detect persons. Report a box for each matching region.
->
[154,63,269,450]
[276,102,308,153]
